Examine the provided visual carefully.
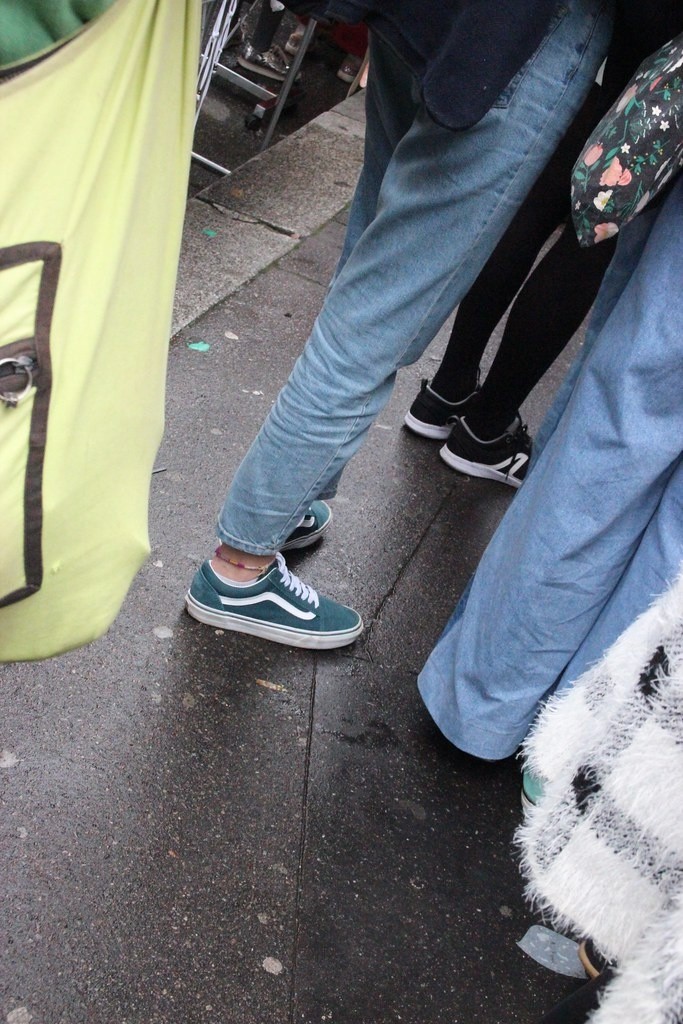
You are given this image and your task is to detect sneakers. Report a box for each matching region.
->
[280,500,332,552]
[238,42,300,82]
[184,551,363,649]
[439,412,533,489]
[404,368,483,441]
[337,54,362,82]
[286,32,317,55]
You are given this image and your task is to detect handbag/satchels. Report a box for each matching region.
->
[569,39,683,248]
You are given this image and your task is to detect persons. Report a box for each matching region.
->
[403,0,683,1024]
[184,0,627,651]
